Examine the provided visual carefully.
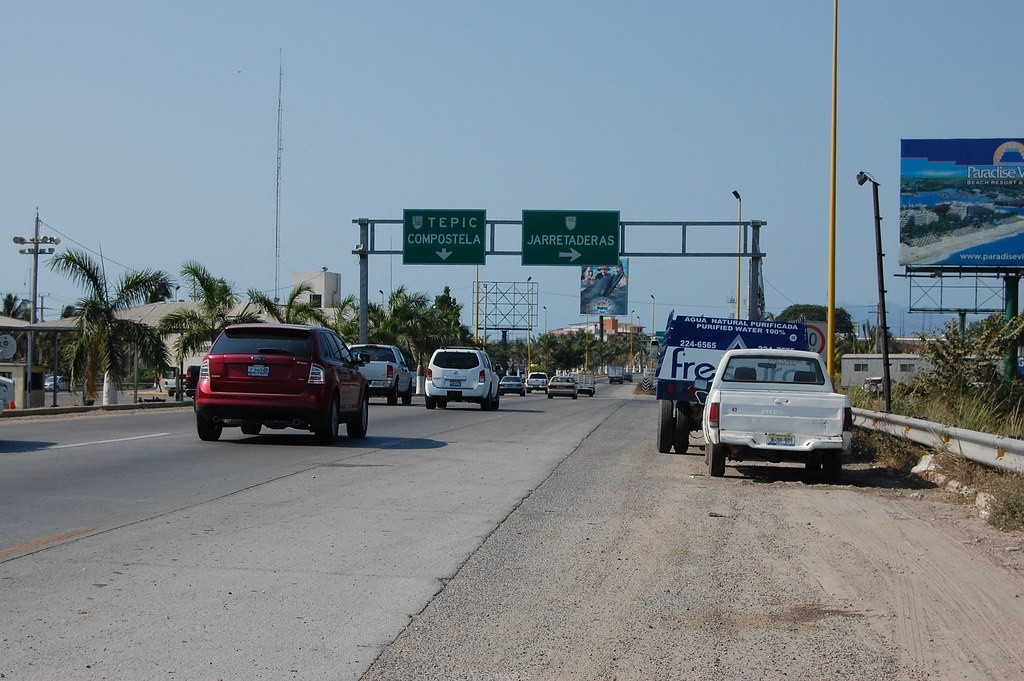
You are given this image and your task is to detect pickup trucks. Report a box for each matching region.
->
[696,349,853,484]
[347,344,412,405]
[525,372,552,394]
[165,374,187,397]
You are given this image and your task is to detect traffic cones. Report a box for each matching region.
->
[9,400,14,408]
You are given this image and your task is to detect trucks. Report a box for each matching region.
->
[572,375,597,397]
[606,365,625,384]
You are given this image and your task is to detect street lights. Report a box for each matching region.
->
[526,276,532,377]
[175,286,180,302]
[630,310,635,366]
[651,295,655,338]
[543,306,547,335]
[733,191,741,321]
[636,316,640,339]
[14,206,60,365]
[379,290,384,309]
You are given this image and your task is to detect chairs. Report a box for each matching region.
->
[732,367,758,381]
[385,354,394,361]
[793,370,817,383]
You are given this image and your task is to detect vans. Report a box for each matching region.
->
[46,375,69,392]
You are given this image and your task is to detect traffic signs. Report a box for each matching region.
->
[522,208,621,266]
[403,209,485,265]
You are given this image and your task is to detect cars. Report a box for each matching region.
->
[184,365,201,397]
[499,376,526,397]
[547,376,579,399]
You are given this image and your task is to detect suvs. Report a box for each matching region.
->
[194,323,371,443]
[424,347,501,411]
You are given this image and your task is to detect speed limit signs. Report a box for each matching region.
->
[806,321,828,361]
